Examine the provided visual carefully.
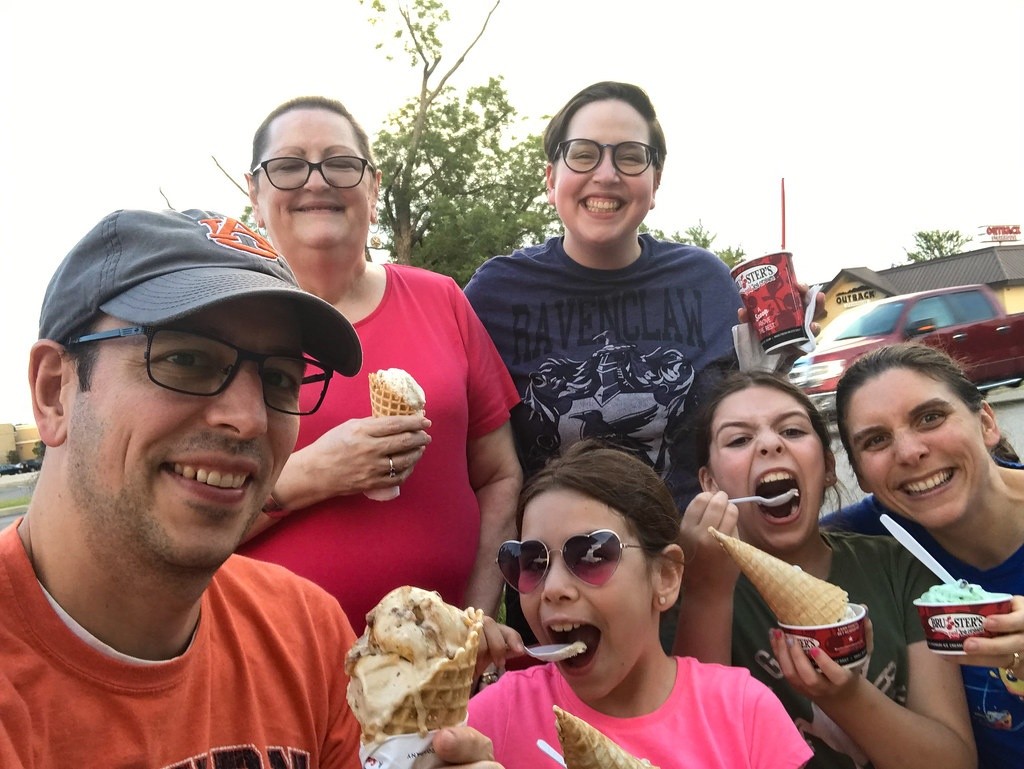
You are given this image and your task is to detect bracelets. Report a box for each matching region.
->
[480,672,500,683]
[261,492,291,519]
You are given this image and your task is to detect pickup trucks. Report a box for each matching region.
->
[783,284,1023,418]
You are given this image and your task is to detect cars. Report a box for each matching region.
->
[0,462,38,475]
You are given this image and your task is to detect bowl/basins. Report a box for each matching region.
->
[777,602,869,673]
[913,592,1014,654]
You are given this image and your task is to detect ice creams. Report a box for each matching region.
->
[552,705,661,769]
[913,579,1013,656]
[343,585,485,748]
[368,367,426,416]
[707,526,857,627]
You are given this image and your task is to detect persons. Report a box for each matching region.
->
[666,359,980,769]
[821,339,1024,769]
[460,80,829,655]
[0,208,506,769]
[467,439,816,769]
[231,96,525,695]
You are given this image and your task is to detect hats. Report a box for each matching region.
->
[39,206,363,378]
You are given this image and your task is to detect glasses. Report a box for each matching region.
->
[251,157,376,191]
[494,529,655,594]
[553,139,660,176]
[55,322,334,418]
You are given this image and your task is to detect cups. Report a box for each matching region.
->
[729,251,810,356]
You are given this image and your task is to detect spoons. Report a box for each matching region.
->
[522,644,586,662]
[728,489,798,507]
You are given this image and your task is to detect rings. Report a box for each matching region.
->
[389,457,396,477]
[1006,653,1020,682]
